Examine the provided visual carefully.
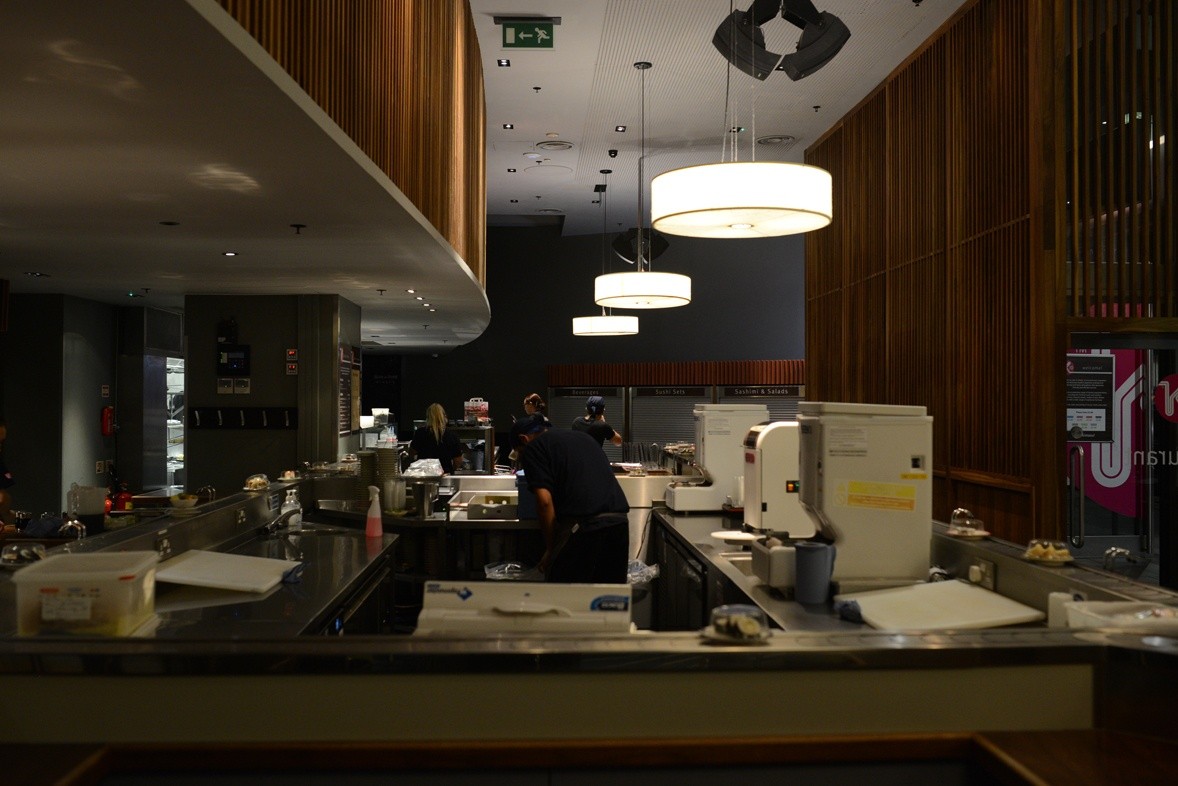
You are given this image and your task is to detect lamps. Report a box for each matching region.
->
[571,182,640,338]
[649,0,835,238]
[592,61,691,309]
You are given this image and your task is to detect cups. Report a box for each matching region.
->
[794,542,837,604]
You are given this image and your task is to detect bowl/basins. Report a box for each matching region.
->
[169,498,199,508]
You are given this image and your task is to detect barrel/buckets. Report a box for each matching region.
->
[372,407,390,425]
[409,481,439,519]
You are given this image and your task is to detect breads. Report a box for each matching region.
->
[246,476,267,489]
[1028,544,1070,559]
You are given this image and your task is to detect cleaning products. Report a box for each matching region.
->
[365,486,383,536]
[280,489,302,526]
[366,536,382,564]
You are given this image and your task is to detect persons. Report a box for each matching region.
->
[410,402,463,513]
[514,414,629,583]
[572,394,622,449]
[498,394,552,473]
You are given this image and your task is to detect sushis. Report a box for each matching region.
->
[713,611,762,639]
[1,546,45,564]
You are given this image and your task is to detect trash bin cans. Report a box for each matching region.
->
[462,449,485,470]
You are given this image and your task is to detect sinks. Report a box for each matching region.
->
[288,528,348,537]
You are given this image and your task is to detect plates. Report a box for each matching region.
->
[166,508,202,517]
[1023,557,1075,566]
[277,477,300,483]
[947,529,991,540]
[1,561,32,571]
[701,626,774,643]
[242,481,270,492]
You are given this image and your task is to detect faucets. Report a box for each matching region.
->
[263,508,303,534]
[58,519,86,540]
[195,485,216,502]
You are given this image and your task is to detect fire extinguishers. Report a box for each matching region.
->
[114,483,133,510]
